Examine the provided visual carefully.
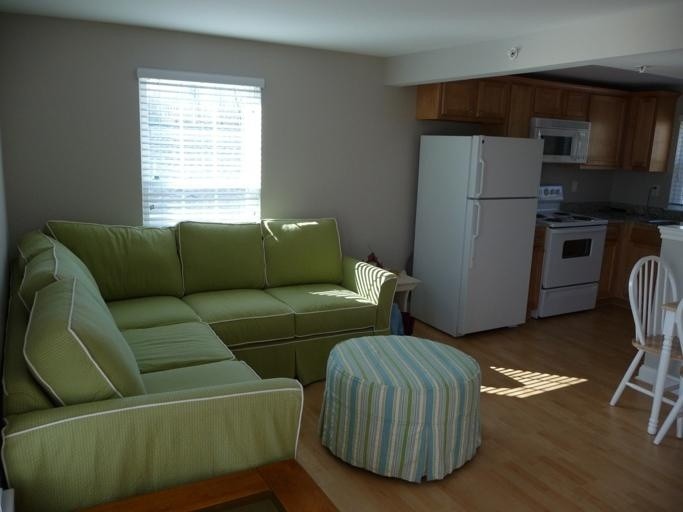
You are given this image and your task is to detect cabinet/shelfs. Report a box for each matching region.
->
[627,90,679,174]
[490,84,531,137]
[532,84,589,117]
[597,223,660,306]
[416,77,505,123]
[579,95,623,172]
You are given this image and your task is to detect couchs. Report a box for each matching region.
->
[0,220,400,512]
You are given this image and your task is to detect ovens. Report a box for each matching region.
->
[536,223,608,319]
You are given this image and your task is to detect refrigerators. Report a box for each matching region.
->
[409,133,547,340]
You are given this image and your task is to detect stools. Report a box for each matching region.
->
[319,333,482,483]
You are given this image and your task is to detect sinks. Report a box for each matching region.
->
[648,219,683,226]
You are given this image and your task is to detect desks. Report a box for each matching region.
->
[647,300,682,438]
[82,456,343,512]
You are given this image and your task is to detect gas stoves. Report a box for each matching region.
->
[534,208,609,229]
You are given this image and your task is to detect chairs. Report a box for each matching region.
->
[654,295,683,446]
[609,253,683,447]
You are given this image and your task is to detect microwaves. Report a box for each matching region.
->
[529,117,590,166]
